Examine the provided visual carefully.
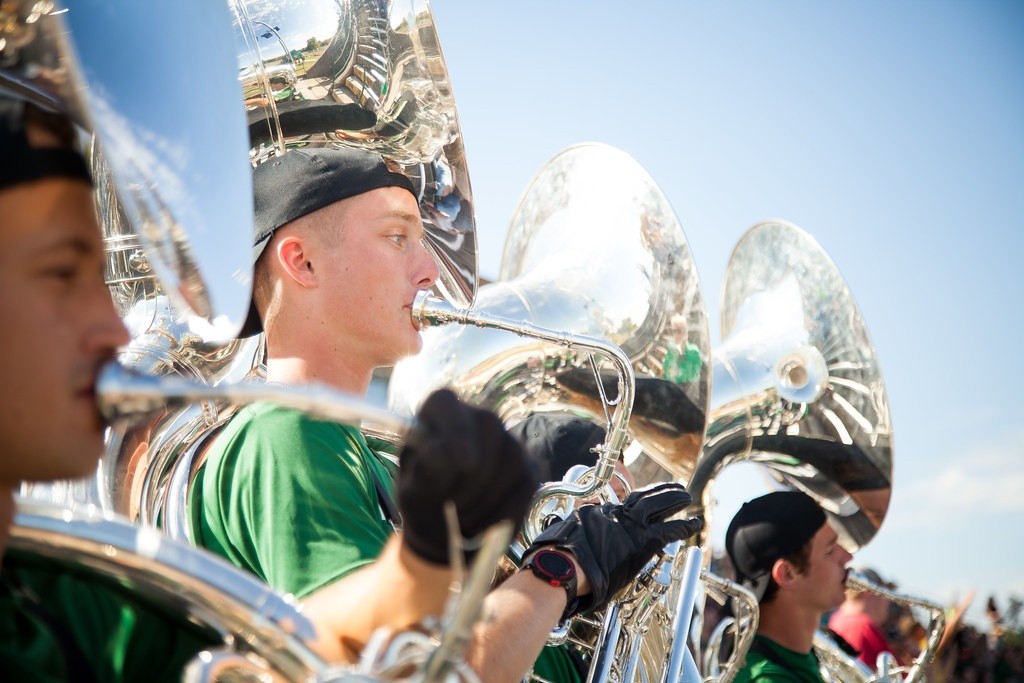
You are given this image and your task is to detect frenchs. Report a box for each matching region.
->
[0,0,968,683]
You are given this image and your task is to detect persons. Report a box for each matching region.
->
[826,567,1024,683]
[0,70,853,683]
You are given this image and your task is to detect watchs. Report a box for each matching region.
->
[518,549,580,620]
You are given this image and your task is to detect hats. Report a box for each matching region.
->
[234,146,419,338]
[507,408,624,484]
[0,69,95,191]
[720,492,828,617]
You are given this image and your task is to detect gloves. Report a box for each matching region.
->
[395,387,537,569]
[520,480,705,619]
